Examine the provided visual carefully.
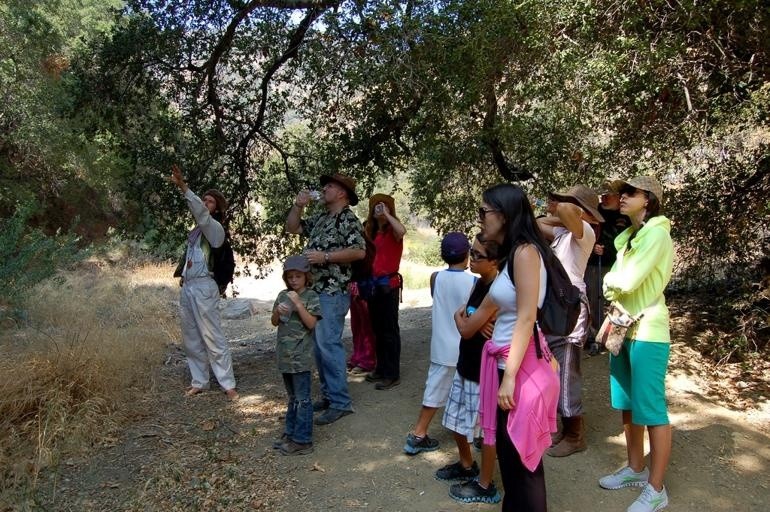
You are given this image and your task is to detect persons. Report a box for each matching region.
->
[404,171,673,512]
[346,221,376,375]
[166,162,240,403]
[363,192,405,391]
[270,255,323,456]
[284,173,368,427]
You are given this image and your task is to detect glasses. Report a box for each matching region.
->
[469,250,491,262]
[478,207,493,219]
[618,186,647,197]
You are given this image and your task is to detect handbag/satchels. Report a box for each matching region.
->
[211,238,235,286]
[507,239,582,337]
[358,278,389,300]
[595,305,635,357]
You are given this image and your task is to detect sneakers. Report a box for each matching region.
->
[227,391,240,400]
[316,409,352,425]
[449,481,501,504]
[187,388,206,394]
[627,484,668,511]
[274,435,313,455]
[311,400,329,409]
[437,461,479,482]
[599,465,649,490]
[472,438,482,450]
[404,433,440,454]
[346,362,400,389]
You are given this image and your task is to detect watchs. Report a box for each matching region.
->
[324,252,329,264]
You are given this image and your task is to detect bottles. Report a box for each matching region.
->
[375,205,383,215]
[278,295,294,323]
[298,191,325,203]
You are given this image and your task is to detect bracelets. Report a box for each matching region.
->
[294,202,303,208]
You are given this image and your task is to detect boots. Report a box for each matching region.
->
[545,415,587,457]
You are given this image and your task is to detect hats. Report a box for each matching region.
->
[203,188,225,210]
[368,193,396,217]
[549,185,606,223]
[318,173,359,206]
[606,176,663,204]
[283,255,311,273]
[441,232,469,262]
